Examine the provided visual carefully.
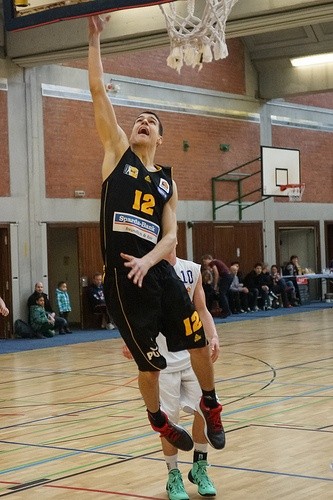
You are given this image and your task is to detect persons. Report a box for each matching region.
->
[88,14,226,451]
[226,262,299,314]
[121,234,221,500]
[30,294,55,337]
[285,255,302,306]
[201,266,221,312]
[87,273,115,330]
[203,255,234,318]
[54,281,72,335]
[28,282,67,330]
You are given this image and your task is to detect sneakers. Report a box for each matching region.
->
[166,468,189,500]
[194,396,227,450]
[58,328,72,335]
[106,323,116,330]
[148,412,193,451]
[188,460,218,497]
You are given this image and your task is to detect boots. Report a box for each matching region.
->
[289,287,299,304]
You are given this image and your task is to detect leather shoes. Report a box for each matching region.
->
[283,302,292,308]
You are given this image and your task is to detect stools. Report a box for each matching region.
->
[274,293,284,308]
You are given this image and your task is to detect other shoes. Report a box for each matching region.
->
[235,306,278,314]
